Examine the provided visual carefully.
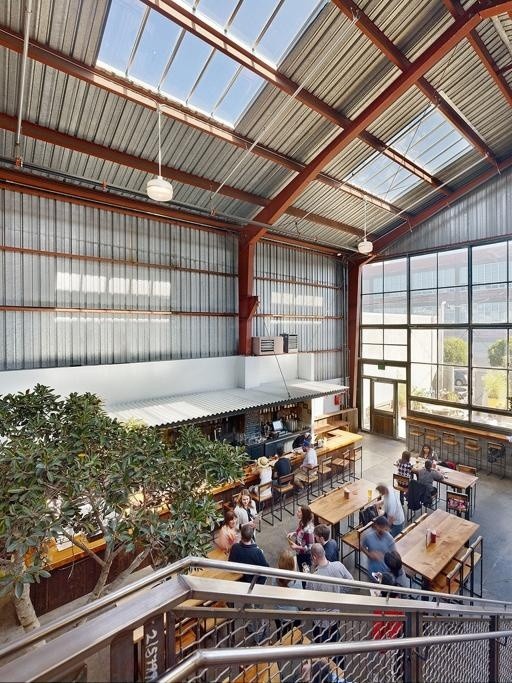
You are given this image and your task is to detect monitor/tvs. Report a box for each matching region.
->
[272,420,283,431]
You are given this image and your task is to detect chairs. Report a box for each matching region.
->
[393,424,506,616]
[165,445,374,655]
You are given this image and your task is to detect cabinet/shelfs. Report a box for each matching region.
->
[314,408,352,435]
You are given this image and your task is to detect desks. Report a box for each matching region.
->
[235,627,345,683]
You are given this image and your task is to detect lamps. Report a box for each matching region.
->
[146,105,173,202]
[358,198,373,254]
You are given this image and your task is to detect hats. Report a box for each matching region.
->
[371,516,388,524]
[299,433,312,446]
[257,456,270,468]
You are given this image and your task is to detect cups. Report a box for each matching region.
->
[368,488,373,497]
[302,562,310,573]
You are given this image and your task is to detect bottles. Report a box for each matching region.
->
[432,460,440,472]
[275,453,278,460]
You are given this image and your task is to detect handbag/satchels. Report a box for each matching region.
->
[359,503,378,530]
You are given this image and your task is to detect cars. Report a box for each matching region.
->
[432,368,470,399]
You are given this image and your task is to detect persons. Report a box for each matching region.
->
[369,482,404,536]
[247,433,321,497]
[218,490,409,670]
[396,444,444,499]
[295,660,351,683]
